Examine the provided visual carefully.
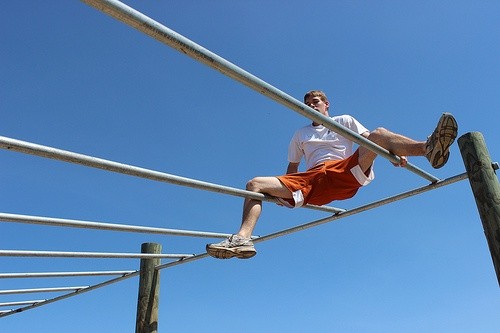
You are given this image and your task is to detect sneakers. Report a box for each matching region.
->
[424,112,458,169]
[206,234,257,259]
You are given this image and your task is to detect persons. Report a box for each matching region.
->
[205,90,459,260]
